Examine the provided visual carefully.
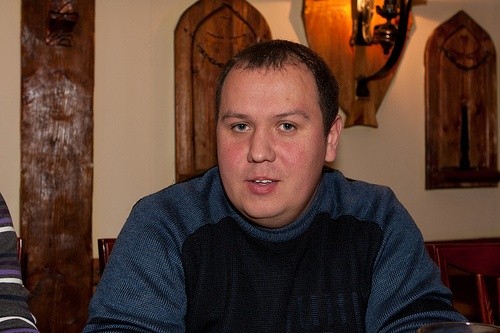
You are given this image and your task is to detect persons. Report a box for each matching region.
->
[83,41,473,333]
[0,192,39,333]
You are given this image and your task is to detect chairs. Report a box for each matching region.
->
[435,243,500,327]
